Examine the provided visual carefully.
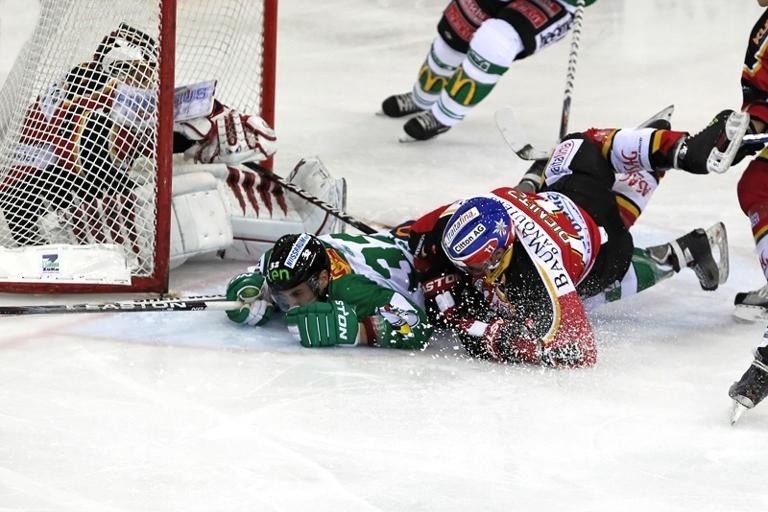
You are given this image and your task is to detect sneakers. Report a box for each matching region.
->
[673,107,734,175]
[728,345,768,412]
[403,108,451,141]
[734,281,768,307]
[674,228,719,292]
[383,91,425,118]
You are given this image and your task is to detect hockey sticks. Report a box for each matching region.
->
[495,107,768,161]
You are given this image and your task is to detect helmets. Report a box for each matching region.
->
[92,23,158,76]
[439,196,516,279]
[266,233,331,291]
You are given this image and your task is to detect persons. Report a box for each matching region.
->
[225,223,717,350]
[728,0,768,409]
[409,109,734,364]
[0,24,346,278]
[384,0,597,142]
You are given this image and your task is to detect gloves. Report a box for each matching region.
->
[731,114,768,167]
[419,270,470,330]
[281,299,358,348]
[456,306,540,366]
[225,272,274,326]
